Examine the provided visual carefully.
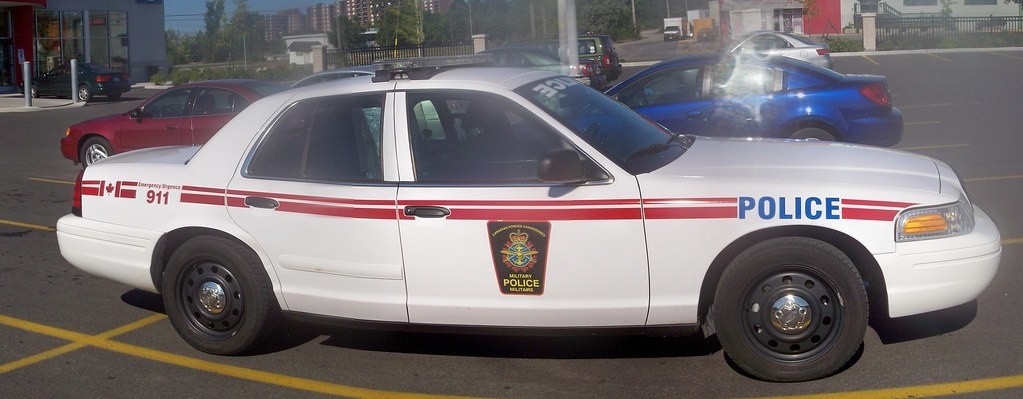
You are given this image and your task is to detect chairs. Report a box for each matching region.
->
[654,69,704,105]
[228,94,243,112]
[462,103,513,158]
[579,43,588,54]
[196,94,216,115]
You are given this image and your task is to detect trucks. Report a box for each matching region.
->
[664,17,688,41]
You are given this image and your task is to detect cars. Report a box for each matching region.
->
[473,48,608,90]
[19,62,132,103]
[291,64,383,89]
[54,54,1001,383]
[680,31,834,83]
[60,78,306,171]
[697,28,715,41]
[510,52,904,149]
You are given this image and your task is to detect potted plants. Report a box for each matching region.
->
[876,26,886,33]
[889,22,901,35]
[975,19,1006,32]
[843,21,856,34]
[906,26,920,34]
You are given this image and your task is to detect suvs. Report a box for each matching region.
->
[503,34,624,86]
